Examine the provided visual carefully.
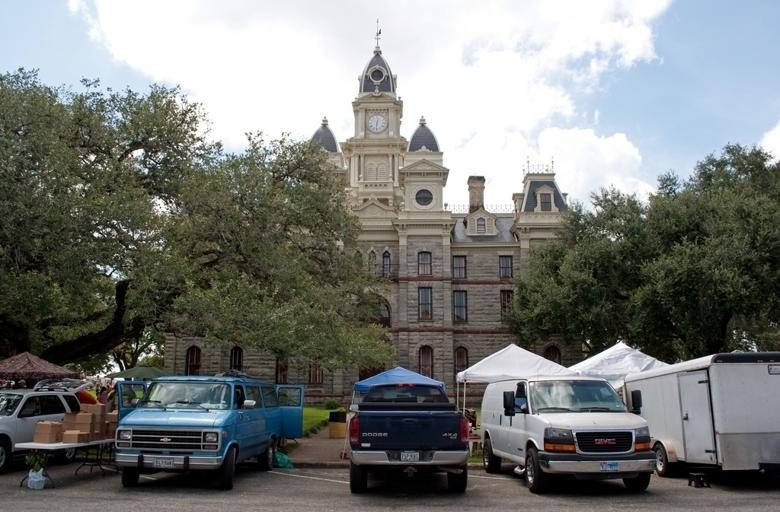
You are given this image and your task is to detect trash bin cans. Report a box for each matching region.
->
[329,411,346,439]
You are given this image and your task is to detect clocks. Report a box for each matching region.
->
[366,113,388,134]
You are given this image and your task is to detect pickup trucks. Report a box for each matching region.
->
[346,383,470,496]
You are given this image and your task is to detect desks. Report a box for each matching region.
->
[15,439,115,489]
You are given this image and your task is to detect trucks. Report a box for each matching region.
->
[623,353,779,477]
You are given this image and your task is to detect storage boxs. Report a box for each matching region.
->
[33,404,118,444]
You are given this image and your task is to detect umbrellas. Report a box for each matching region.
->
[105,365,169,395]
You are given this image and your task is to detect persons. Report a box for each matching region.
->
[95,375,136,413]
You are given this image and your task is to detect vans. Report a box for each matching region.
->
[115,376,302,490]
[480,376,657,494]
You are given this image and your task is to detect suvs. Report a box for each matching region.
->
[0,374,94,467]
